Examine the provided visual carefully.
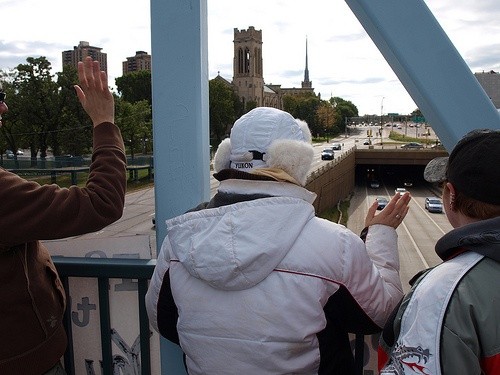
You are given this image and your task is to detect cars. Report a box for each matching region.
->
[430,142,445,150]
[321,148,335,161]
[364,139,371,146]
[394,188,408,198]
[5,149,26,160]
[332,144,342,151]
[401,142,425,150]
[367,168,375,177]
[376,196,389,211]
[369,179,380,189]
[349,120,430,137]
[404,180,414,188]
[424,196,444,214]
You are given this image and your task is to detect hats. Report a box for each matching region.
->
[213,107,314,186]
[424,129,500,207]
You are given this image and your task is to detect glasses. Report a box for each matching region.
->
[0,92,6,104]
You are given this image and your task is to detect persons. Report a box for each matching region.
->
[378,129,500,375]
[0,56,125,373]
[144,107,411,375]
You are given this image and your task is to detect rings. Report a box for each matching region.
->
[395,214,401,219]
